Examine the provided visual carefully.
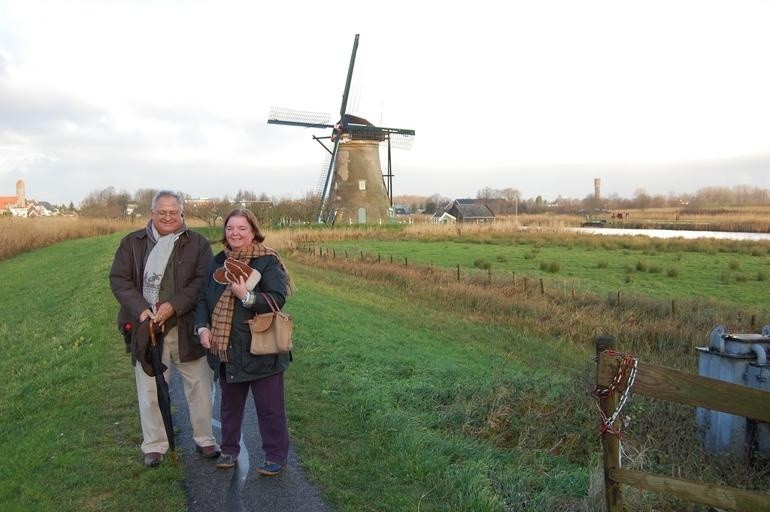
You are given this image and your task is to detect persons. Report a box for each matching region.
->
[110,191,222,467]
[193,208,292,474]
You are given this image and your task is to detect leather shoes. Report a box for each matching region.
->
[144,453,164,466]
[195,444,222,457]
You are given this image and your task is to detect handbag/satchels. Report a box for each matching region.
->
[245,292,293,354]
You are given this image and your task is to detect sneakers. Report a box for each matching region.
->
[215,454,238,467]
[256,461,284,474]
